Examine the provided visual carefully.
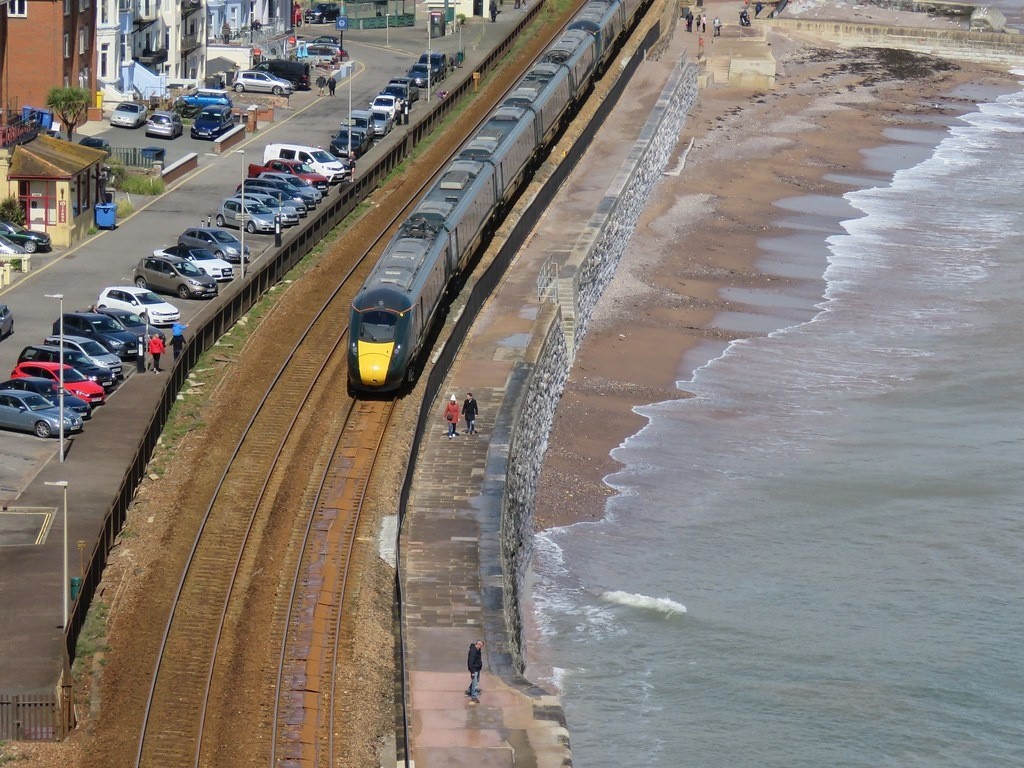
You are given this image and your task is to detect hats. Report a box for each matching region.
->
[450,394,456,401]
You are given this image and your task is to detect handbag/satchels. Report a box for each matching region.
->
[447,414,453,420]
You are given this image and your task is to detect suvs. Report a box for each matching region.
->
[306,2,340,25]
[419,53,448,77]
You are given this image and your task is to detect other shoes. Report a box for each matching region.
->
[467,432,470,436]
[466,688,472,696]
[453,433,455,438]
[472,431,475,435]
[449,435,451,438]
[471,697,479,702]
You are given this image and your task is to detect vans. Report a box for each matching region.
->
[263,143,347,184]
[246,60,312,90]
[339,110,377,143]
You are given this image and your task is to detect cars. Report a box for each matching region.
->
[294,35,348,66]
[329,130,368,159]
[78,136,112,161]
[132,226,249,300]
[0,285,180,438]
[0,218,52,256]
[174,88,235,142]
[214,158,330,234]
[144,110,185,140]
[109,101,150,129]
[0,302,14,338]
[232,70,296,96]
[367,61,437,137]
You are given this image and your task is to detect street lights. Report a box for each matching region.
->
[43,293,64,462]
[423,10,431,102]
[386,13,389,46]
[235,149,245,279]
[44,480,69,631]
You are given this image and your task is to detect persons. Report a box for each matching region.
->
[169,333,186,360]
[699,36,704,52]
[438,89,449,101]
[465,639,484,703]
[376,9,382,17]
[739,10,751,26]
[89,305,98,313]
[755,0,762,16]
[148,333,166,376]
[442,394,461,439]
[316,74,336,98]
[695,14,701,33]
[339,150,357,195]
[685,11,694,32]
[395,98,403,125]
[462,393,479,436]
[701,14,706,32]
[173,319,190,336]
[713,16,722,37]
[489,0,526,23]
[222,19,262,44]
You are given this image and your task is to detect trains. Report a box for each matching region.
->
[346,0,650,400]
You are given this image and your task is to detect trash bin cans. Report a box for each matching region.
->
[95,203,116,229]
[142,147,165,170]
[96,92,105,108]
[71,577,82,600]
[22,107,54,130]
